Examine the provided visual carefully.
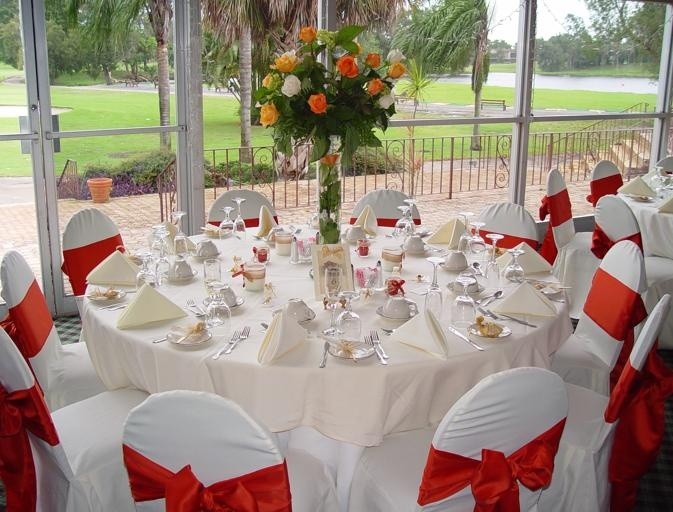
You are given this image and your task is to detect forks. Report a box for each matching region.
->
[364,330,390,365]
[212,326,251,361]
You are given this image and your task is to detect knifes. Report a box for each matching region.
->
[319,342,330,368]
[448,326,484,351]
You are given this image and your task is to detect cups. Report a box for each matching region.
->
[171,227,309,322]
[344,225,479,319]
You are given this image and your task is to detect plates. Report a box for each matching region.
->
[164,230,316,345]
[87,292,127,302]
[340,228,563,338]
[630,196,653,202]
[325,340,375,360]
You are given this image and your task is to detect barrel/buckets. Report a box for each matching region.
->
[86,177,112,203]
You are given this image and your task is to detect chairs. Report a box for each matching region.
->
[589,159,623,208]
[655,156,672,173]
[207,190,278,228]
[350,364,570,512]
[0,326,156,511]
[121,389,336,512]
[544,168,592,270]
[471,201,540,250]
[349,189,421,227]
[593,193,673,353]
[554,239,645,383]
[62,208,124,320]
[1,251,104,411]
[566,292,671,512]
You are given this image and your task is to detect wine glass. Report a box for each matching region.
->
[651,166,673,205]
[323,198,525,342]
[136,197,247,335]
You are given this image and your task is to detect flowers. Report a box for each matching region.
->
[251,25,407,241]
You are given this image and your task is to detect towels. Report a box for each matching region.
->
[616,176,655,200]
[657,195,673,213]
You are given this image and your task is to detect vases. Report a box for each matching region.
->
[315,134,340,243]
